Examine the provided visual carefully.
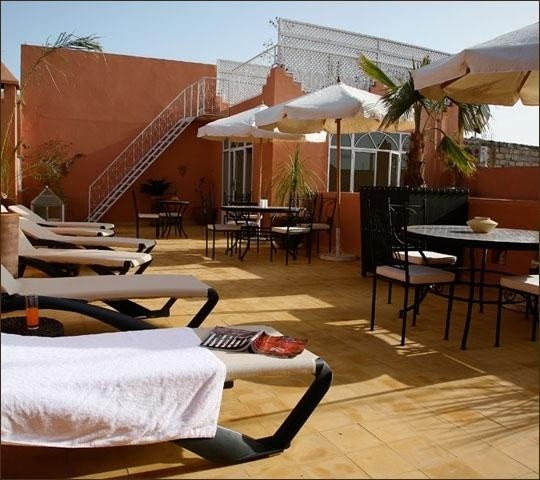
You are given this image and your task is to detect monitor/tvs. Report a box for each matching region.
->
[198,324,308,358]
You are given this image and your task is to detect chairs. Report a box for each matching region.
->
[495,275,539,348]
[369,193,456,346]
[2,205,157,278]
[202,194,336,265]
[131,192,159,238]
[0,264,218,331]
[1,324,330,463]
[388,196,457,327]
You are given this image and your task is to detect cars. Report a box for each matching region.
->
[25,295,40,330]
[260,199,268,208]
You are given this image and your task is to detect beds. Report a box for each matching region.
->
[254,74,418,252]
[193,101,327,239]
[411,21,539,107]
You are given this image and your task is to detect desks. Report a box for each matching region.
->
[402,225,538,351]
[160,200,190,238]
